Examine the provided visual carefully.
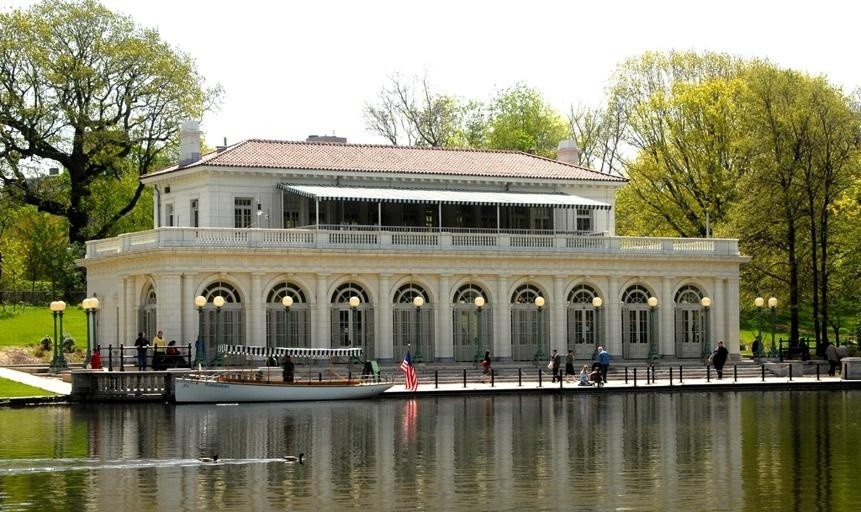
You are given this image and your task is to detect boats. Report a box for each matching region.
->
[172,343,395,402]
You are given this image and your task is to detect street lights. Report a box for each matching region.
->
[753,296,782,360]
[699,297,714,361]
[348,295,360,365]
[280,295,295,364]
[646,295,662,361]
[212,294,227,366]
[534,297,548,364]
[590,297,605,360]
[413,295,426,367]
[193,295,208,368]
[473,296,487,363]
[49,296,99,368]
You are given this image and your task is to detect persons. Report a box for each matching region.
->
[135,332,150,370]
[751,336,848,376]
[151,331,166,370]
[91,348,103,369]
[482,351,490,374]
[282,355,294,382]
[165,340,185,364]
[551,347,609,386]
[713,342,728,378]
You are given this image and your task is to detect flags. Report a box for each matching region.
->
[400,351,418,392]
[402,399,417,434]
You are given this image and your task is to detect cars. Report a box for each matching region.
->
[841,339,858,346]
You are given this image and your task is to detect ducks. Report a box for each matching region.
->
[282,452,304,463]
[198,454,220,463]
